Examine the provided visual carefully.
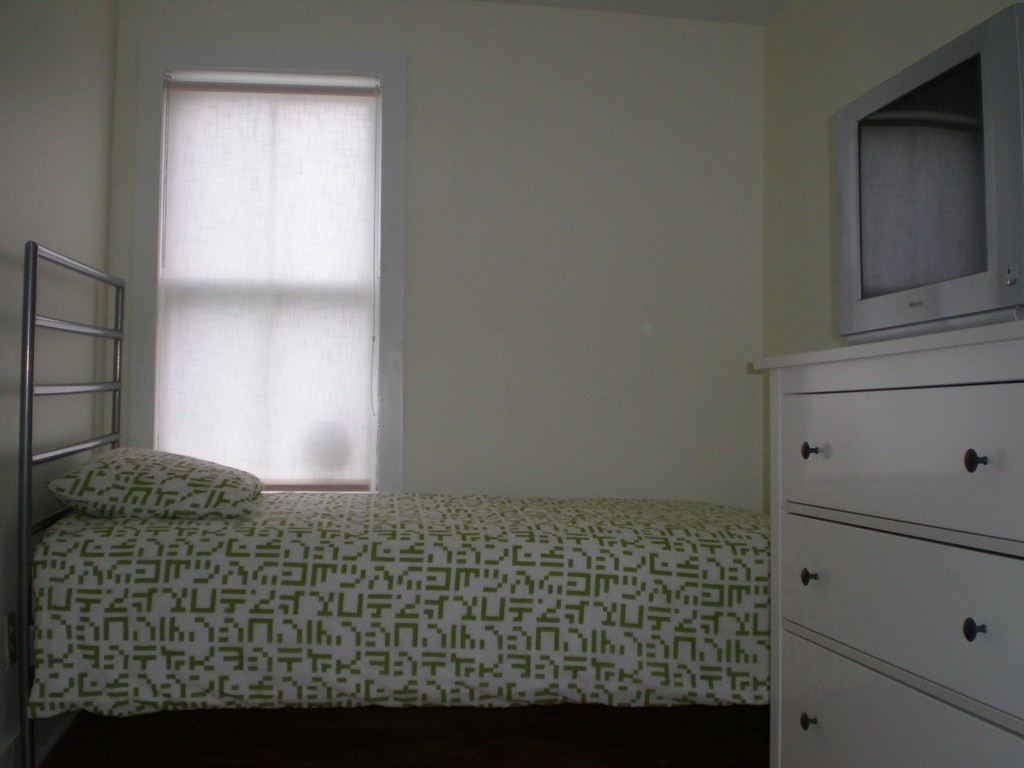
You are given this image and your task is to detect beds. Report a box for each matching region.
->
[14,233,774,768]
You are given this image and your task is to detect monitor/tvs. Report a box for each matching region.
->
[831,2,1023,347]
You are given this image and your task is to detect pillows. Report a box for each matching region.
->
[43,445,265,527]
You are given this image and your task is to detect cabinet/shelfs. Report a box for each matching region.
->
[749,317,1024,768]
